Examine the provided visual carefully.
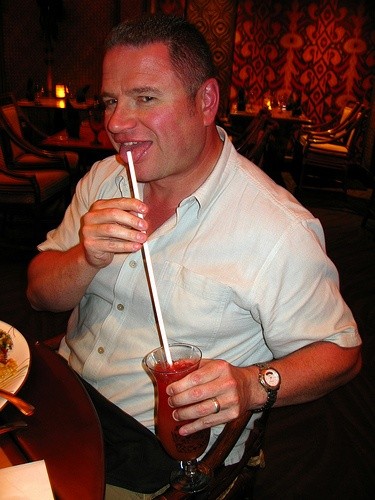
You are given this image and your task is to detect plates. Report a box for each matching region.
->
[0,321,30,414]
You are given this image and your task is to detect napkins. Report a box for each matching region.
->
[0,458,55,500]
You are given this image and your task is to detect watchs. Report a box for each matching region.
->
[249,362,281,412]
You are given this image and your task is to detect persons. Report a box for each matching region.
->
[25,31,364,500]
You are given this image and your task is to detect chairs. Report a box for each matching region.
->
[221,105,365,211]
[0,105,80,225]
[40,332,269,500]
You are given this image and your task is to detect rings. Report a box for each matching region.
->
[211,397,220,413]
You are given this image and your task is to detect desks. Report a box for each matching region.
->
[41,120,111,162]
[0,320,104,500]
[229,110,312,151]
[17,96,95,109]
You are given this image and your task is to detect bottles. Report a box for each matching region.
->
[286,91,303,116]
[237,87,245,111]
[26,75,34,101]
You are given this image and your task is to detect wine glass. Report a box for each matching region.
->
[144,343,213,493]
[32,80,74,104]
[248,91,285,114]
